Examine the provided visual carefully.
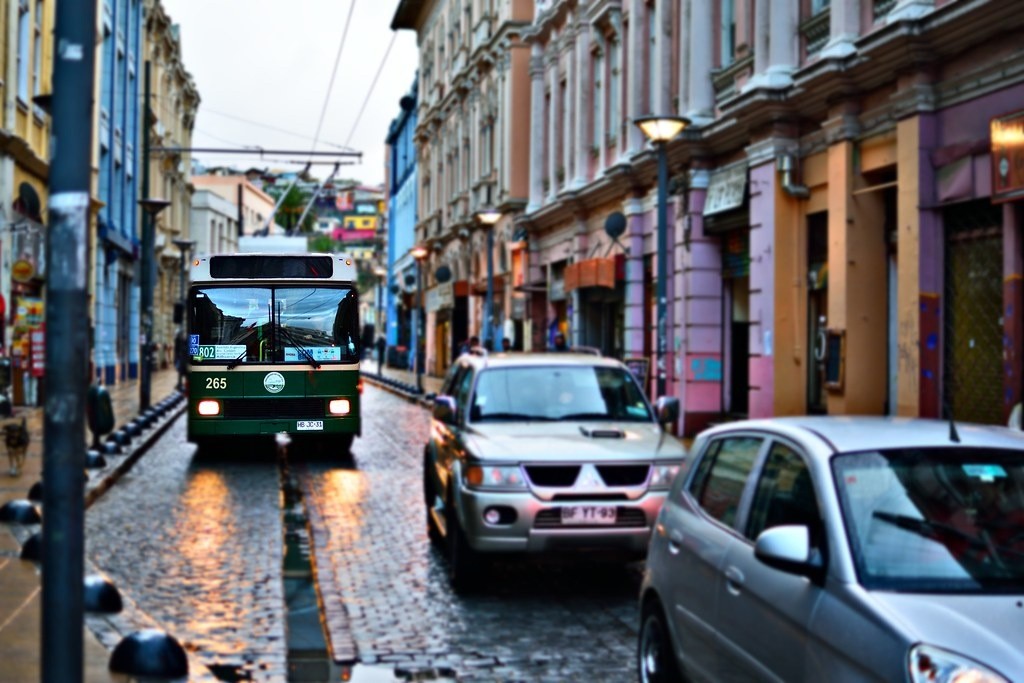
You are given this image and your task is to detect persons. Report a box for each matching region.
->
[501,338,513,352]
[552,333,571,353]
[460,336,480,353]
[302,316,346,347]
[483,340,494,352]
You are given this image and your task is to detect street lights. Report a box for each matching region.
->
[411,246,428,389]
[475,202,501,352]
[634,114,690,397]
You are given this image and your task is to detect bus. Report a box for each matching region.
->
[174,235,375,451]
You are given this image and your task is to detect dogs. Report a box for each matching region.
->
[2,416,31,475]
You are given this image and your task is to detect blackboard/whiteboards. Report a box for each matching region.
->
[824,335,841,383]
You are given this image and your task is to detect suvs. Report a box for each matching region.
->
[422,345,688,592]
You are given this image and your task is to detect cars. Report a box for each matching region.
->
[637,414,1024,683]
